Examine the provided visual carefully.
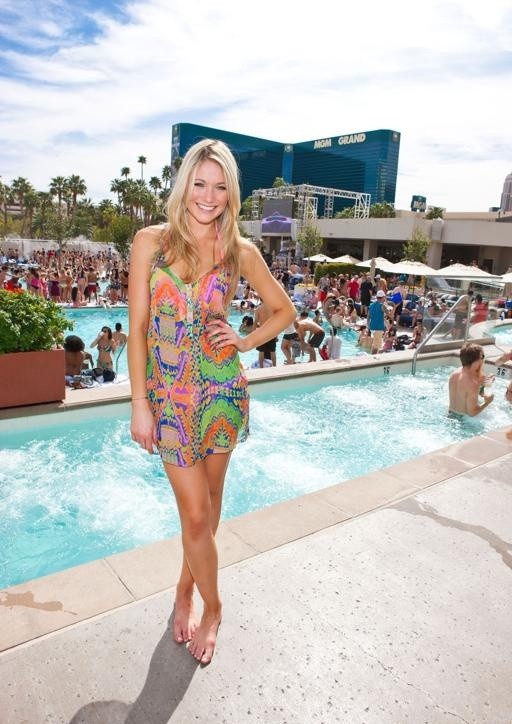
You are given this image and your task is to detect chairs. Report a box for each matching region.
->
[353,291,461,328]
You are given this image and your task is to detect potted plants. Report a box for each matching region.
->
[0,289,76,409]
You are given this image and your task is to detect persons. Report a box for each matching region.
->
[124,137,298,666]
[2,246,131,389]
[447,344,496,416]
[234,248,425,366]
[491,350,512,405]
[423,286,512,340]
[475,345,496,387]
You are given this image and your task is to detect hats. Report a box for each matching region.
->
[376,290,386,298]
[374,274,381,278]
